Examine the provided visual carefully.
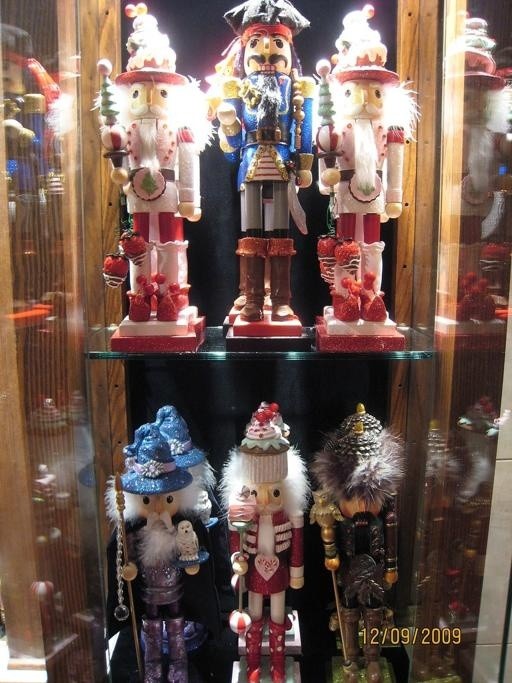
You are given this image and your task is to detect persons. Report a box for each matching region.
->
[209,1,313,320]
[0,20,60,311]
[98,0,417,325]
[310,402,404,683]
[103,401,404,683]
[103,405,217,683]
[222,402,310,683]
[457,10,503,322]
[107,2,209,323]
[316,3,420,324]
[440,393,498,623]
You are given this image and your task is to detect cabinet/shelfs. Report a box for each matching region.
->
[0,0,512,683]
